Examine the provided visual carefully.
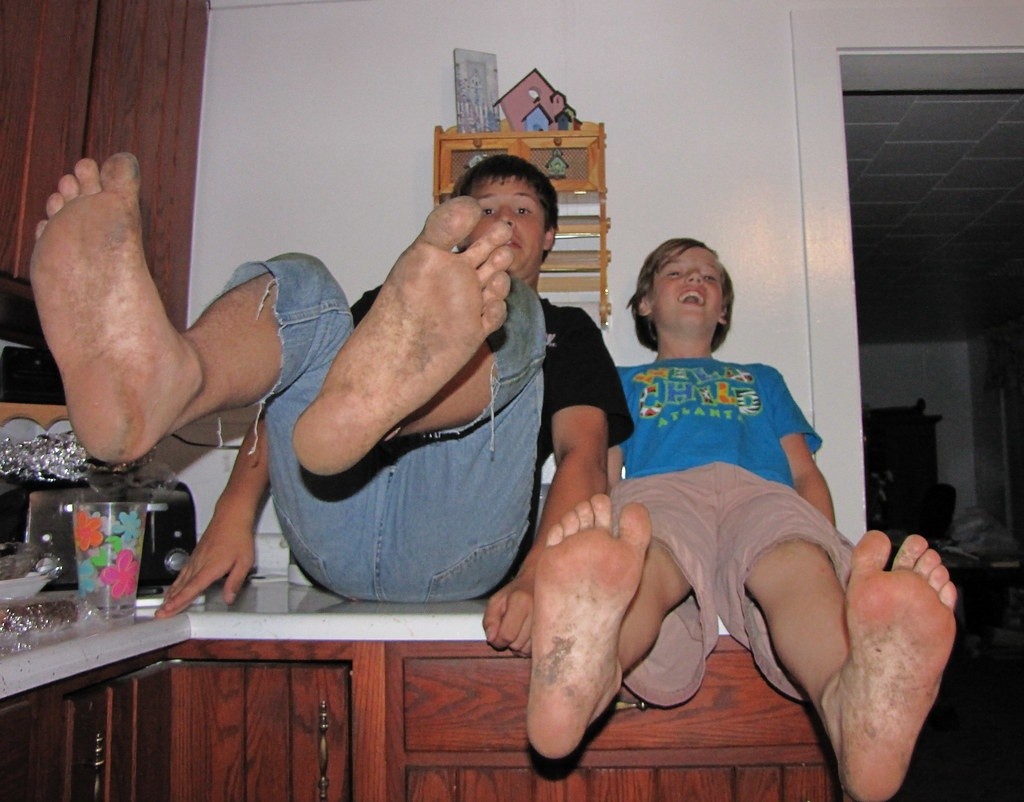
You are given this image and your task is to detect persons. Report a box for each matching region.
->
[28,153,633,659]
[526,239,958,802]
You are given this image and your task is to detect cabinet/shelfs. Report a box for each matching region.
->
[0,0,209,333]
[1,637,844,802]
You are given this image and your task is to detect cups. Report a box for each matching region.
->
[71,501,147,616]
